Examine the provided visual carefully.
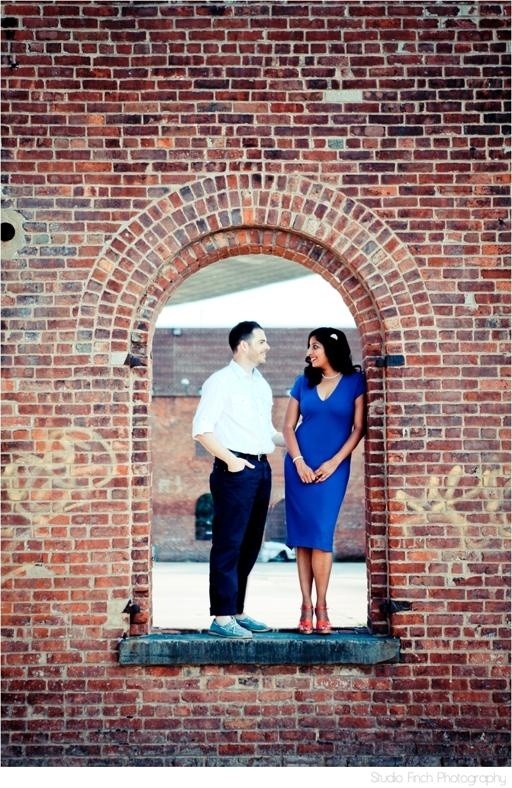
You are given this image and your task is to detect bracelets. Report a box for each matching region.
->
[292,456,303,463]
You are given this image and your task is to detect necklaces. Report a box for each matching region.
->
[322,372,341,379]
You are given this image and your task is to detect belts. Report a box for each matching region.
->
[239,453,267,462]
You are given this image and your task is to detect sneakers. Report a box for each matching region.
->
[236,616,272,632]
[208,617,253,639]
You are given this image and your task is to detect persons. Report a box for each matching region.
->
[191,321,275,641]
[285,327,367,635]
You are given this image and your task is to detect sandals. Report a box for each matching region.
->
[299,606,314,634]
[315,608,332,634]
